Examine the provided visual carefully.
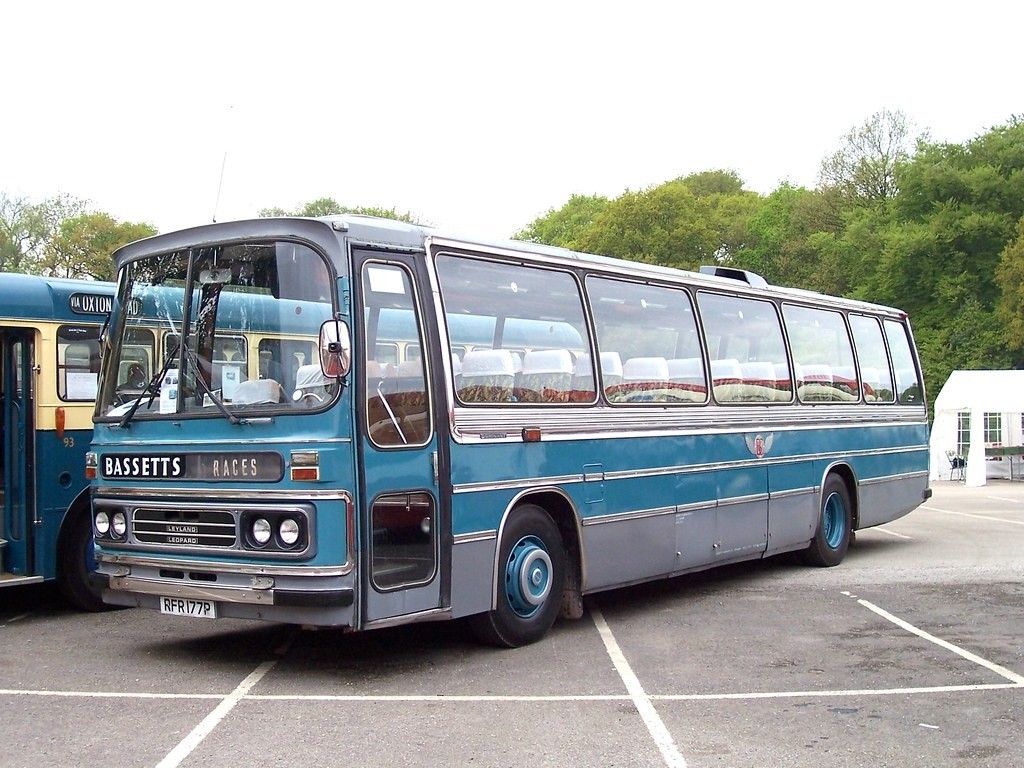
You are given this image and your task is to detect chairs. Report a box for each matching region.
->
[233,378,280,404]
[945,450,967,483]
[295,350,900,406]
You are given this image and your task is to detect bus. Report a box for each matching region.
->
[82,217,936,647]
[0,272,589,616]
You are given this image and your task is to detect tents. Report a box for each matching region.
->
[929,369,1024,488]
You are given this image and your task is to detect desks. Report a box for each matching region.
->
[985,446,1024,481]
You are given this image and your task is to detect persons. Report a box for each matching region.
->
[112,363,152,407]
